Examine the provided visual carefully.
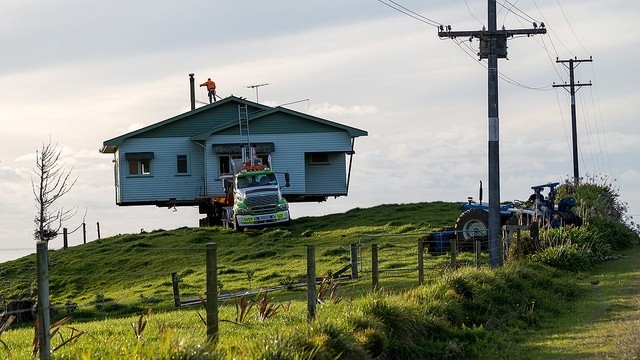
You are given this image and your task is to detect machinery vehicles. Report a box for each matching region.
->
[221,164,290,232]
[422,180,583,257]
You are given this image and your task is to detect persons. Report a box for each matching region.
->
[524,188,545,205]
[200,78,216,103]
